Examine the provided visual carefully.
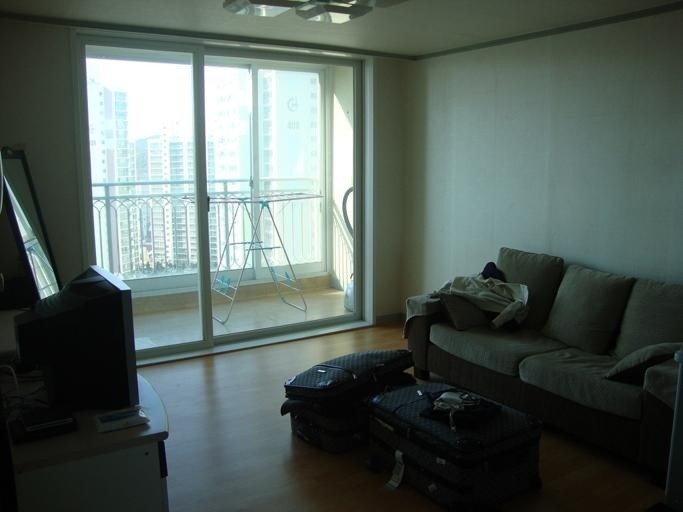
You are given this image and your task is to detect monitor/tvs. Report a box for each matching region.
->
[14,265,139,413]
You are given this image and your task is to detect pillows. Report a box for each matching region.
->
[496,246,683,383]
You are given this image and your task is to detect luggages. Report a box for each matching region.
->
[283,349,416,455]
[365,381,543,512]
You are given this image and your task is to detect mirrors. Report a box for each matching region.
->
[1,146,63,299]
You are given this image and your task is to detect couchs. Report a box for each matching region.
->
[406,276,679,476]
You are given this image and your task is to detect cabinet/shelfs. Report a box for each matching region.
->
[0,372,169,512]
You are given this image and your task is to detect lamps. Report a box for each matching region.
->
[223,0,409,25]
